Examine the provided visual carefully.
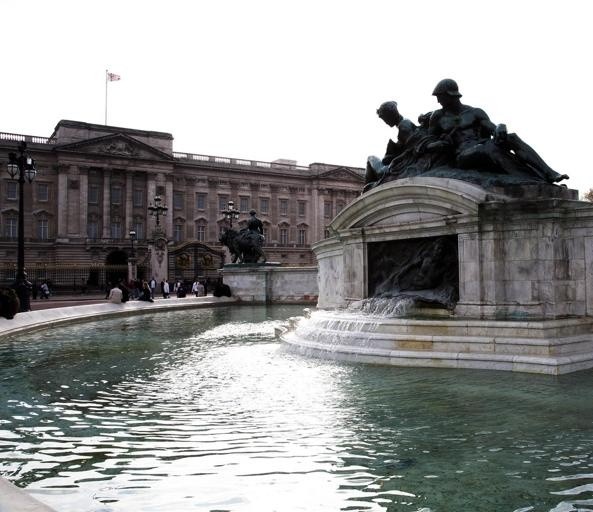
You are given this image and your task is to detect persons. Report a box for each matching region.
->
[39,279,53,299]
[79,278,87,294]
[425,78,569,183]
[395,239,457,291]
[363,101,418,185]
[105,277,232,303]
[240,209,263,235]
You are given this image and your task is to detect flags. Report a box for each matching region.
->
[108,73,120,81]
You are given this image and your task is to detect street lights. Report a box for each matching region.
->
[6,140,38,311]
[147,192,168,237]
[127,226,136,260]
[221,199,239,229]
